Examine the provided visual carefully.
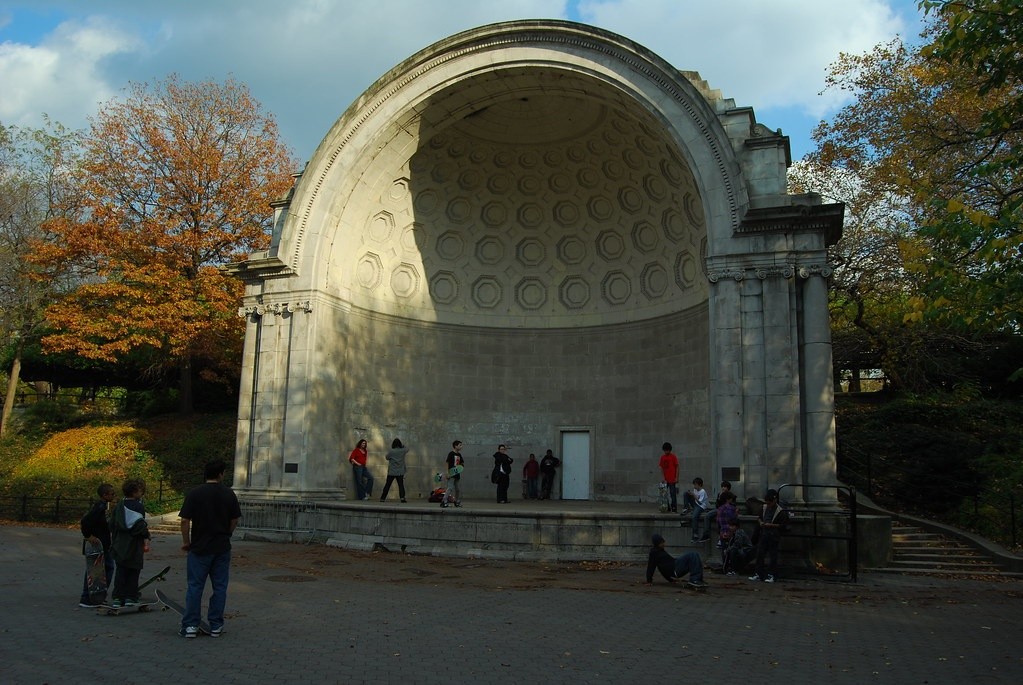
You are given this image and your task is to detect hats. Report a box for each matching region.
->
[651,534,664,545]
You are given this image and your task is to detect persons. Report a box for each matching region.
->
[178,460,242,638]
[681,477,709,543]
[657,442,679,513]
[380,438,409,504]
[724,518,753,577]
[646,533,710,587]
[748,489,789,583]
[699,481,739,567]
[540,449,560,501]
[523,453,540,500]
[107,478,152,607]
[78,483,115,607]
[847,376,856,391]
[349,439,374,501]
[440,440,464,508]
[493,444,513,504]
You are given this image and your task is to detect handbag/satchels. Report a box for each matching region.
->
[491,470,500,483]
[751,526,763,545]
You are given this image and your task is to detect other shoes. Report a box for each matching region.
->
[701,582,709,587]
[682,507,691,515]
[686,581,703,587]
[440,500,449,508]
[497,501,507,504]
[399,498,407,503]
[689,535,700,543]
[699,535,711,542]
[453,500,462,508]
[725,570,736,576]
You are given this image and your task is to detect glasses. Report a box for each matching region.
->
[106,492,115,496]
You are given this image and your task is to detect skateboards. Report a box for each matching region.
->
[656,480,669,512]
[154,587,211,635]
[680,579,711,594]
[84,538,108,604]
[138,565,171,591]
[99,599,160,615]
[521,479,527,497]
[434,465,464,483]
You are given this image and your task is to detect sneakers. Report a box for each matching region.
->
[211,625,223,638]
[177,625,198,637]
[748,573,760,582]
[113,599,124,607]
[77,600,100,608]
[124,598,141,606]
[764,574,774,583]
[99,600,109,607]
